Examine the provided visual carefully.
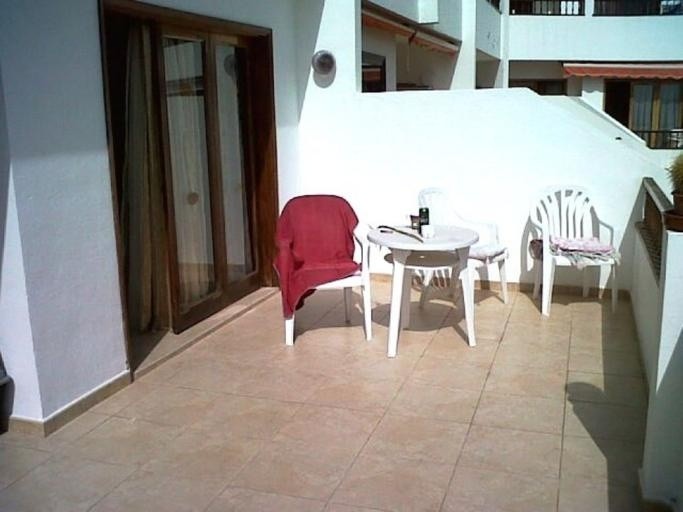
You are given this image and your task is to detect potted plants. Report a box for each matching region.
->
[662,152,683,232]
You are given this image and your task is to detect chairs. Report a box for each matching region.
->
[418,187,510,310]
[530,186,618,317]
[286,195,371,345]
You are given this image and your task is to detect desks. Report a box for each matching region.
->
[367,222,479,358]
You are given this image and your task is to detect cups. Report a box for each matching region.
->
[422,225,437,241]
